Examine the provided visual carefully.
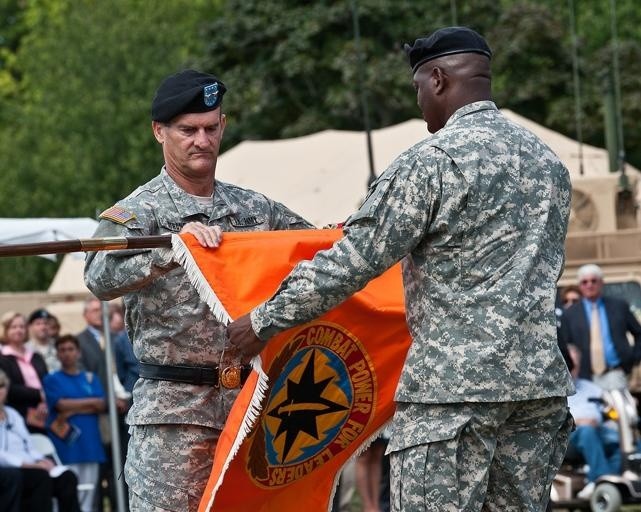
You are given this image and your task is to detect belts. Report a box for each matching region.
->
[135,362,255,388]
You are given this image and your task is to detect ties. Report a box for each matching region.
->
[589,303,606,375]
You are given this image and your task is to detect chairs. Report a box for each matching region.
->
[30,433,94,508]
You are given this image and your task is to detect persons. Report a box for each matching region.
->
[224,25,579,511]
[1,293,144,512]
[561,343,632,502]
[80,69,323,511]
[557,283,584,311]
[561,263,641,454]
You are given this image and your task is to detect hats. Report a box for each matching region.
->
[28,309,49,324]
[151,69,226,123]
[408,25,492,74]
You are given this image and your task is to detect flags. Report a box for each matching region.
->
[165,226,416,511]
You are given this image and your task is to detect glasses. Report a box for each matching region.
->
[562,298,578,303]
[581,279,596,283]
